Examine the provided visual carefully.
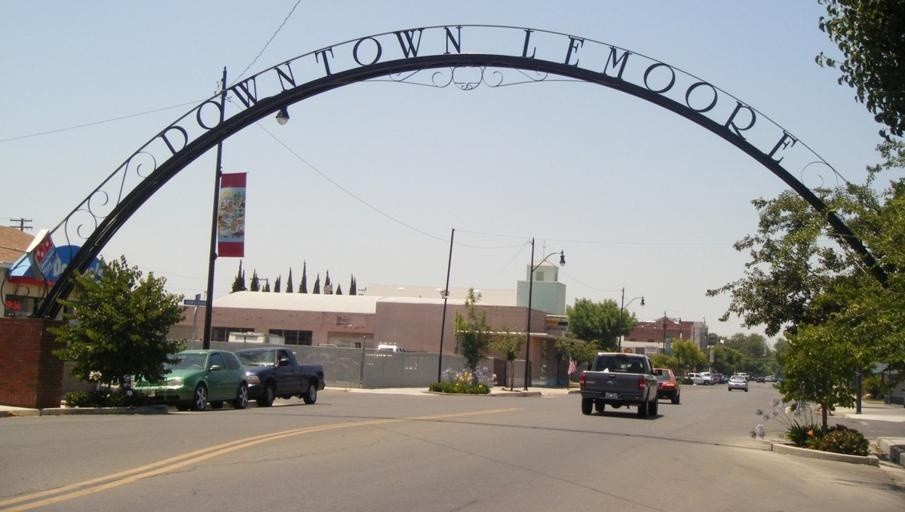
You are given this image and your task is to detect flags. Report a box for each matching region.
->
[567,357,576,376]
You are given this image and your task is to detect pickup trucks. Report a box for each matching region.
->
[233,347,326,407]
[580,351,659,419]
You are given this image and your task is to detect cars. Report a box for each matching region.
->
[651,366,681,403]
[686,370,750,391]
[757,375,777,383]
[130,348,249,411]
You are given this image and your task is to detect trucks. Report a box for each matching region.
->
[377,344,399,352]
[226,330,286,345]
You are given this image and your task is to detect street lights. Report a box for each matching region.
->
[523,236,568,391]
[619,287,646,352]
